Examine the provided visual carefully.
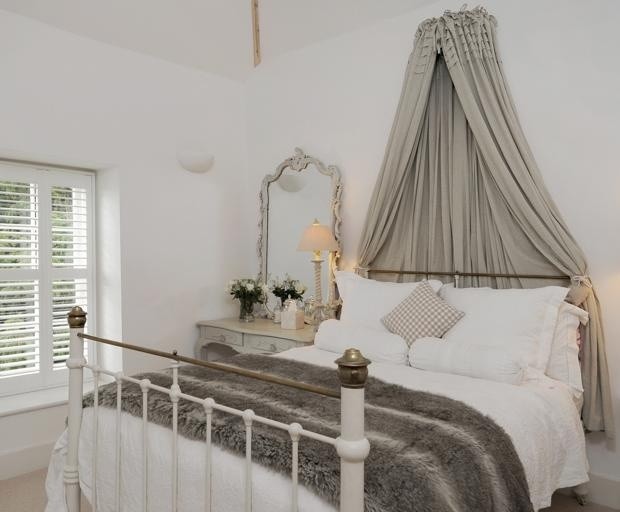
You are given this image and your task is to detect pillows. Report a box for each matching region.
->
[380,278,465,346]
[408,336,528,384]
[315,320,409,364]
[436,282,570,376]
[335,270,442,332]
[544,301,589,398]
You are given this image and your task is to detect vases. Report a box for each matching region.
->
[238,297,254,322]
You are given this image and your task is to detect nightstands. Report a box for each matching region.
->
[194,317,316,364]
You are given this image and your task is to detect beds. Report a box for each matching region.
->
[45,260,589,512]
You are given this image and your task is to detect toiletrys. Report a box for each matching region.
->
[273,300,284,323]
[259,308,268,319]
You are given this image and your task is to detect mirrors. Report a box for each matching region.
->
[255,147,341,318]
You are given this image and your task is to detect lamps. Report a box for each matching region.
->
[297,218,338,326]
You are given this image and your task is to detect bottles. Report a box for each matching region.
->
[304,296,321,325]
[282,294,294,312]
[272,301,282,324]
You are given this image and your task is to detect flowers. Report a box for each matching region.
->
[229,278,269,306]
[271,277,308,306]
